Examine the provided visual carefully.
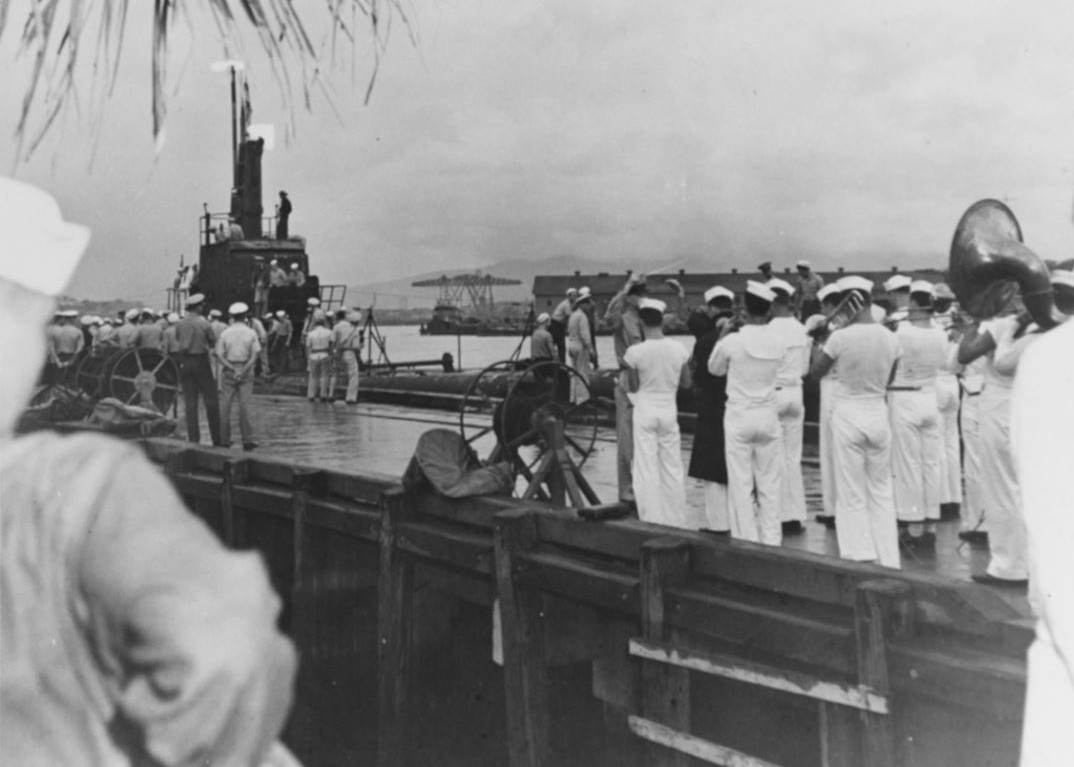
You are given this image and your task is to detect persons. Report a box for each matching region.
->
[174,265,197,289]
[531,286,599,405]
[215,218,244,241]
[0,177,306,767]
[604,262,1074,584]
[1012,315,1073,767]
[301,296,362,406]
[43,292,294,456]
[275,189,291,238]
[269,258,305,287]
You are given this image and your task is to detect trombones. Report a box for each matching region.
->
[808,290,866,341]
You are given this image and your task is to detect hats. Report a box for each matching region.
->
[184,293,205,305]
[575,286,592,304]
[766,279,796,296]
[704,285,735,303]
[882,275,912,292]
[62,311,79,318]
[836,276,873,294]
[637,297,668,314]
[142,308,154,316]
[167,312,179,324]
[746,280,777,303]
[934,282,956,299]
[125,308,139,320]
[346,310,362,323]
[261,312,273,320]
[276,310,286,318]
[307,297,321,306]
[537,313,550,323]
[229,302,248,315]
[210,309,222,317]
[816,283,838,302]
[910,279,933,295]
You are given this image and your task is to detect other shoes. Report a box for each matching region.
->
[972,568,1026,585]
[899,530,936,547]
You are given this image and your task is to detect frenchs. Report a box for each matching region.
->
[948,198,1074,332]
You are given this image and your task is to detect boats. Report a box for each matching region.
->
[413,273,532,336]
[164,63,349,379]
